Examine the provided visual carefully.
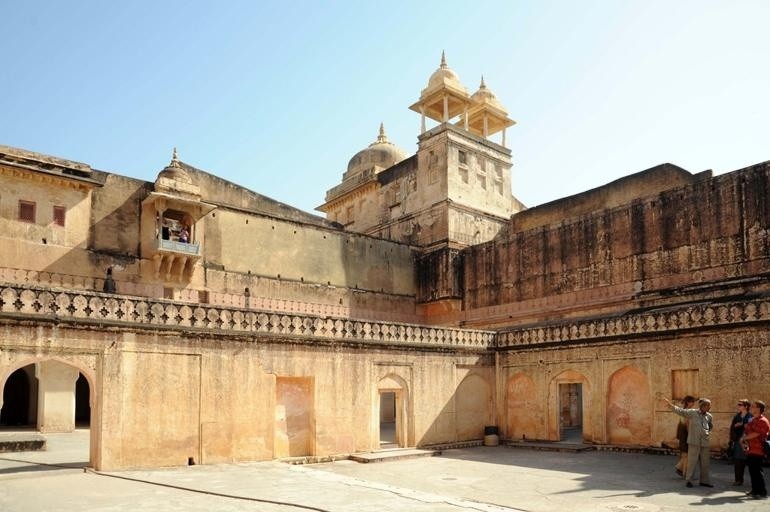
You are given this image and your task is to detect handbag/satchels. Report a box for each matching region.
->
[763,441,770,453]
[677,417,687,441]
[726,441,736,465]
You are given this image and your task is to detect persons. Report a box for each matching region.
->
[741,400,770,498]
[659,394,715,487]
[728,398,757,485]
[157,217,169,240]
[674,395,699,480]
[178,224,189,243]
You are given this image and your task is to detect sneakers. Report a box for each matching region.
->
[699,482,714,487]
[673,466,683,476]
[687,481,693,488]
[747,490,766,497]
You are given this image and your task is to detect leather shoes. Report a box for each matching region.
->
[733,481,743,486]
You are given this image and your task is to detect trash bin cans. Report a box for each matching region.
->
[483,425,499,446]
